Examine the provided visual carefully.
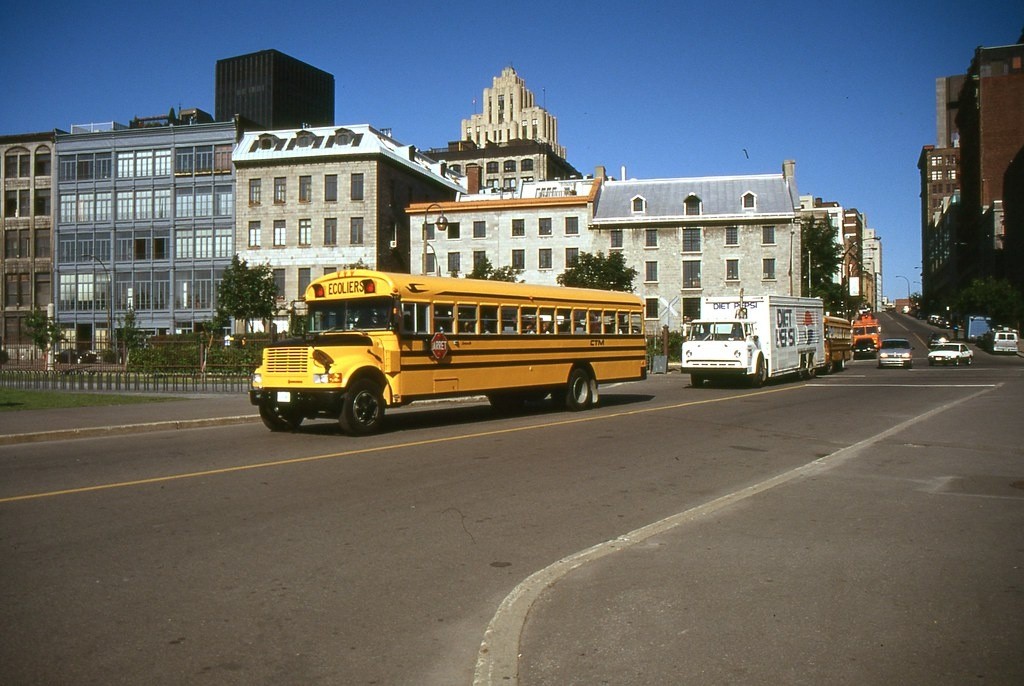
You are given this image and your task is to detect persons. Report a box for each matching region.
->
[402,316,639,334]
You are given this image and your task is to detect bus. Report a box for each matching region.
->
[249,269,649,438]
[821,315,854,375]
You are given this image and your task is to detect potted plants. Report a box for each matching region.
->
[255,200,259,204]
[250,200,254,205]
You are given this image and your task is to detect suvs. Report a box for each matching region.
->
[877,338,915,369]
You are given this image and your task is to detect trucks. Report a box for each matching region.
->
[851,315,882,358]
[985,327,1019,355]
[968,316,992,344]
[681,294,827,390]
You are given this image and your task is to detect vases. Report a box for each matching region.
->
[276,298,284,301]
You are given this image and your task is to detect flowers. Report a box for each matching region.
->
[276,294,284,298]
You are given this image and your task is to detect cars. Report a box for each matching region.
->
[901,305,964,332]
[928,333,951,349]
[927,343,974,367]
[60,351,95,365]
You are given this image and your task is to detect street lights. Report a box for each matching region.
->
[840,236,882,286]
[896,276,910,307]
[80,253,110,352]
[422,203,449,277]
